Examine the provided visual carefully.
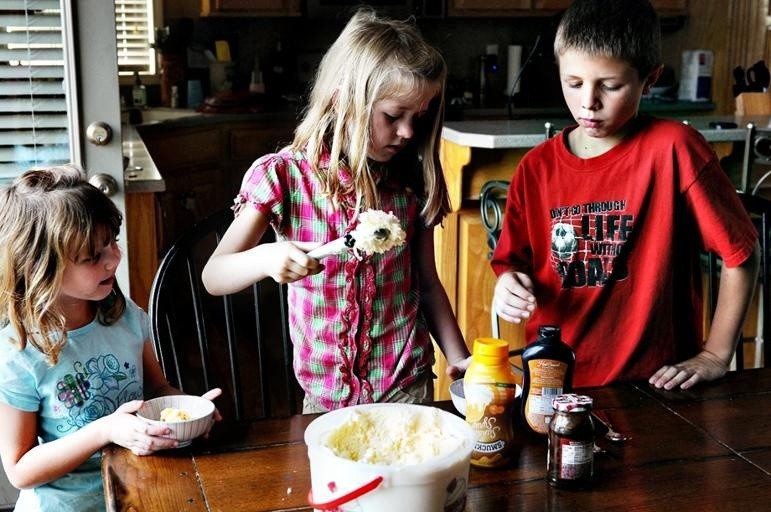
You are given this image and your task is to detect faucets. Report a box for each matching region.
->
[439,33,452,53]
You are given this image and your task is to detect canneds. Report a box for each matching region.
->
[546,394,594,489]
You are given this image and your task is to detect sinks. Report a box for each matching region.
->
[122,106,200,128]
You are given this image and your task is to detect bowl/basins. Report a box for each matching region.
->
[135,394,215,450]
[448,377,523,417]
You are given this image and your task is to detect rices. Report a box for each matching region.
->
[160,408,190,422]
[344,207,408,262]
[325,406,470,465]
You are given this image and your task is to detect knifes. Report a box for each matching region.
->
[732,61,770,98]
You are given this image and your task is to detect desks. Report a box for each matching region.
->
[101,379,771,512]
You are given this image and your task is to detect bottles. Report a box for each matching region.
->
[522,325,576,444]
[462,337,517,469]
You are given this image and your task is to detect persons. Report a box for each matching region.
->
[489,0,761,390]
[0,160,222,512]
[201,5,474,416]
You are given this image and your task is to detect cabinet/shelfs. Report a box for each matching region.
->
[126,193,163,312]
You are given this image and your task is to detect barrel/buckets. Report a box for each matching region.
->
[304,401,478,512]
